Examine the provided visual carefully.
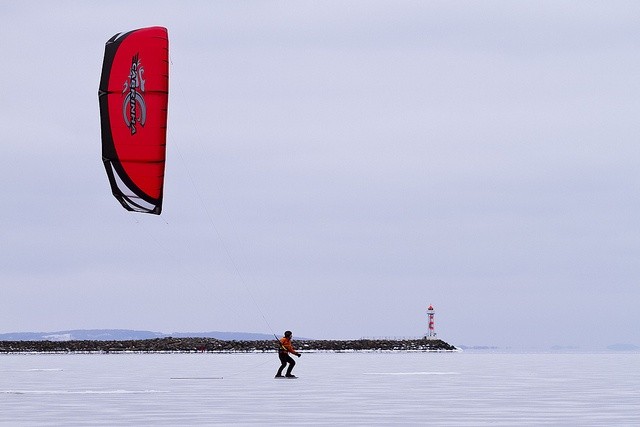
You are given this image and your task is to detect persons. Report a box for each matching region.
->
[275,331,301,377]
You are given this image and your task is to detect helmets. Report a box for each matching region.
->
[283,331,292,338]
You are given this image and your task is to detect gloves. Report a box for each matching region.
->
[296,354,301,357]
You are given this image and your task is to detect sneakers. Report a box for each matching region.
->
[286,374,295,377]
[276,373,284,377]
